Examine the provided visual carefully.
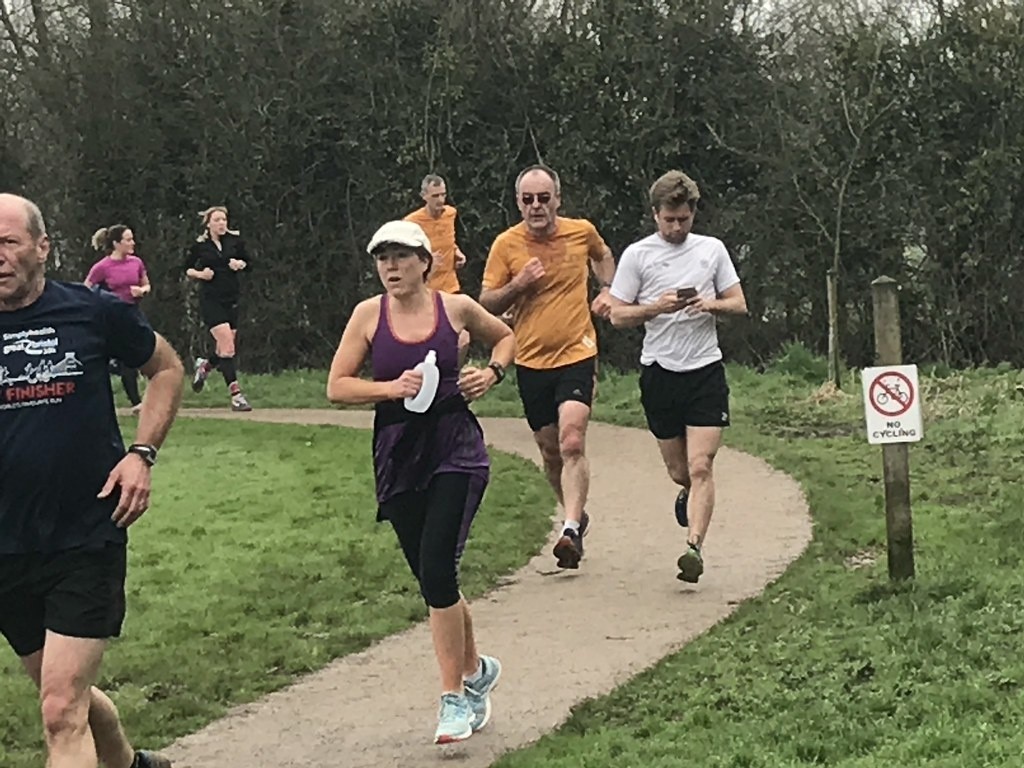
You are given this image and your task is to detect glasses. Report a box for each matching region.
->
[517,193,557,205]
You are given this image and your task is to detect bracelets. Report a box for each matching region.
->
[599,282,611,288]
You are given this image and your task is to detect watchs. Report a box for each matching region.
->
[128,444,158,466]
[489,362,506,384]
[142,288,148,297]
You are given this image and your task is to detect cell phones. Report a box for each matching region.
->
[677,286,696,308]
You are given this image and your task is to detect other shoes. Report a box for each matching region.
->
[676,534,704,583]
[130,403,141,416]
[675,488,690,527]
[191,357,214,393]
[231,393,253,411]
[561,510,589,538]
[552,526,582,569]
[135,749,171,768]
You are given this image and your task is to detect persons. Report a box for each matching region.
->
[183,207,254,412]
[403,173,471,370]
[478,164,616,572]
[610,168,748,583]
[0,192,184,768]
[325,221,518,743]
[84,226,151,414]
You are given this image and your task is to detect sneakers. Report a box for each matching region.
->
[463,655,502,731]
[433,692,476,744]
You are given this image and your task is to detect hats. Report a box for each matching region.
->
[366,220,432,255]
